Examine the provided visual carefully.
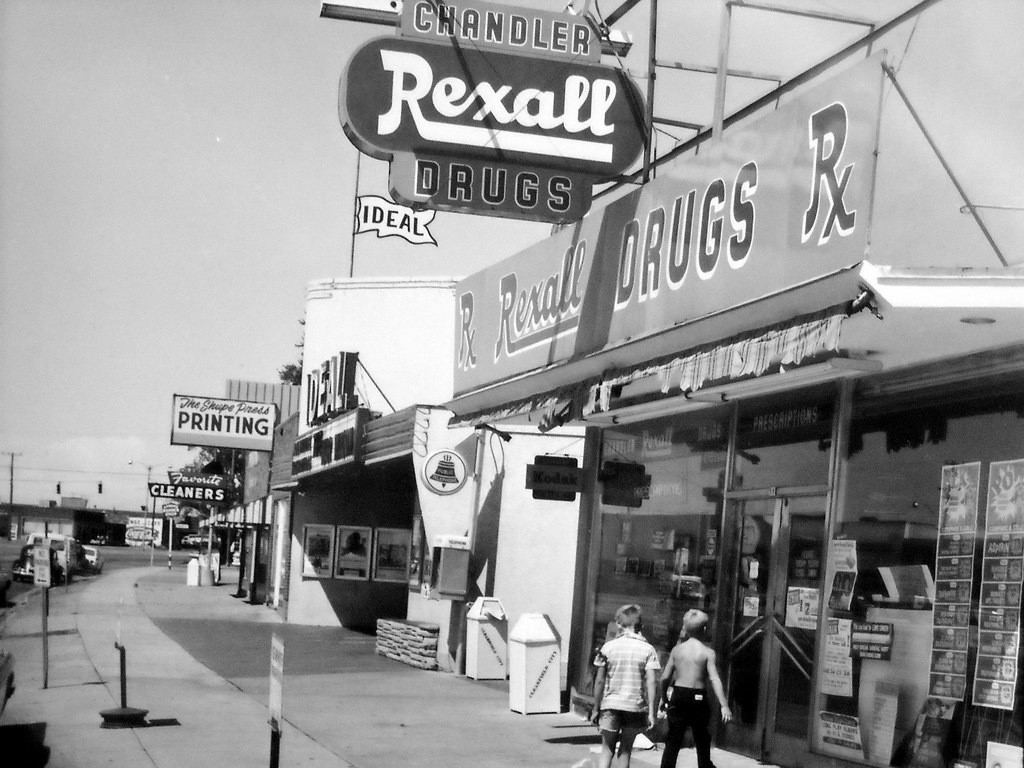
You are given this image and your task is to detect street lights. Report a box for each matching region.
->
[126,460,173,551]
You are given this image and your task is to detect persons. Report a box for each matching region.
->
[591,603,663,768]
[657,607,732,768]
[831,573,852,608]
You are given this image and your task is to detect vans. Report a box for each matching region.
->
[27,534,88,580]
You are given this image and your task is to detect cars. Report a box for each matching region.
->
[82,543,105,574]
[10,542,66,587]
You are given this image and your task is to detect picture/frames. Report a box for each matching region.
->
[333,526,372,581]
[372,529,410,584]
[299,522,335,580]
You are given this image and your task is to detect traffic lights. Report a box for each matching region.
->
[99,483,102,493]
[57,484,60,494]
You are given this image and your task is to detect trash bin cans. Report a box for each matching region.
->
[466,596,508,681]
[509,614,562,716]
[187,558,201,586]
